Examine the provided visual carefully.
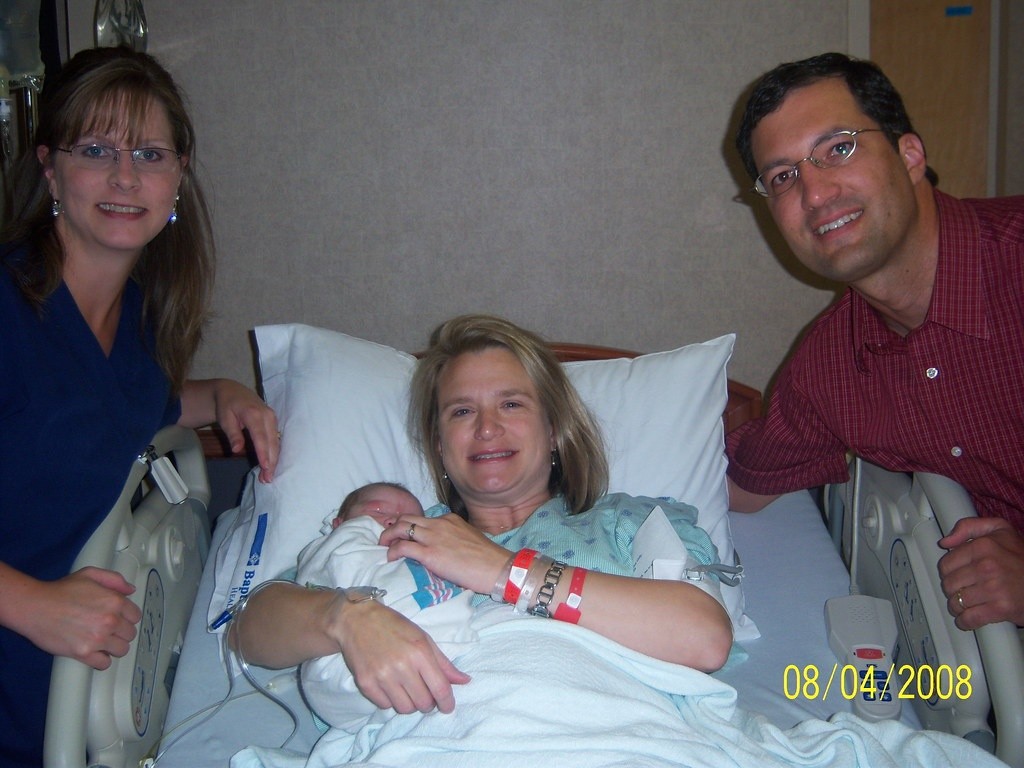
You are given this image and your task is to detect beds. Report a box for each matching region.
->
[44,322,1024,768]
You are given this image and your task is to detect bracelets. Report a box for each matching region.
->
[491,548,588,625]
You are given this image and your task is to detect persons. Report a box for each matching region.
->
[729,54,1024,631]
[228,313,733,718]
[0,43,284,768]
[292,481,478,733]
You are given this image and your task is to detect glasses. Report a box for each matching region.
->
[55,143,182,173]
[748,127,903,198]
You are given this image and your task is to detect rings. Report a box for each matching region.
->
[277,432,281,438]
[409,523,415,541]
[957,591,968,610]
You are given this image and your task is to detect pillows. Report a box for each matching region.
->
[204,322,761,641]
